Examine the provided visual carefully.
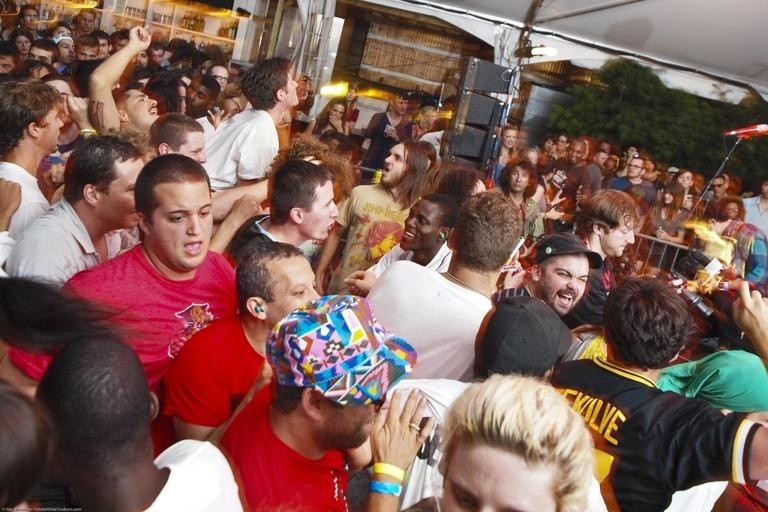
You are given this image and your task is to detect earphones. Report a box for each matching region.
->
[254,306,266,316]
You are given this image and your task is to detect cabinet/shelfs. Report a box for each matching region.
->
[110,0,240,59]
[16,0,105,30]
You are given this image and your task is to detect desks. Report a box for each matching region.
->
[633,232,698,273]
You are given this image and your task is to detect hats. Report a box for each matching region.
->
[536,231,602,270]
[265,294,417,407]
[52,33,73,44]
[474,296,572,372]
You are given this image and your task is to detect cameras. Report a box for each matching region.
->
[665,270,715,318]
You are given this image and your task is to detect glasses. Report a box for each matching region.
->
[726,207,738,213]
[711,184,722,188]
[22,15,38,19]
[628,163,643,169]
[212,75,229,83]
[370,393,387,413]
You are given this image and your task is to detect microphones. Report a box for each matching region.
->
[721,123,767,140]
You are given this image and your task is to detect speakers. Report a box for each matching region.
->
[447,121,503,158]
[450,89,509,131]
[462,56,517,91]
[447,154,497,181]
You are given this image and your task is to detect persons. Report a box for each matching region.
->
[1,1,768,512]
